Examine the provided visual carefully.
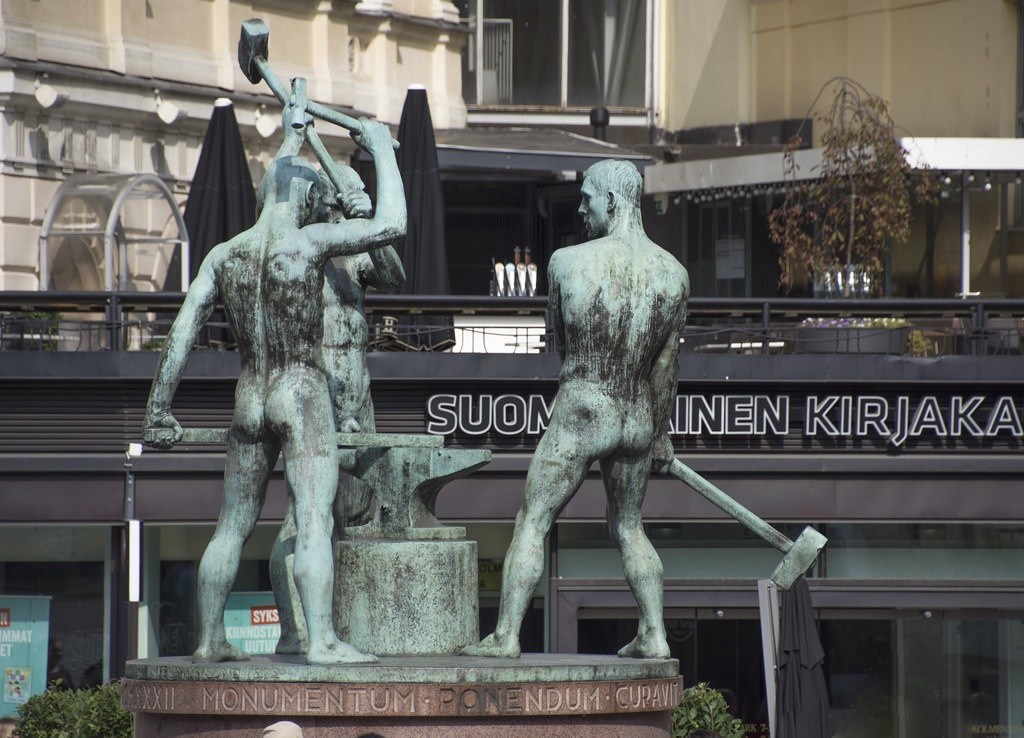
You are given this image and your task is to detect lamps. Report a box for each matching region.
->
[34,78,57,108]
[256,107,276,138]
[153,88,179,125]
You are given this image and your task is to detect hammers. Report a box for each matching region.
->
[670,456,828,590]
[237,18,395,285]
[289,76,401,151]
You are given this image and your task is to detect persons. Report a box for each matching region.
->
[139,117,411,663]
[255,103,406,654]
[453,159,690,658]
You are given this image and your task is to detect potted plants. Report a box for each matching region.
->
[767,71,934,358]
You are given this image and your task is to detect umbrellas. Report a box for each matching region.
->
[776,578,833,738]
[367,82,457,356]
[152,100,255,344]
